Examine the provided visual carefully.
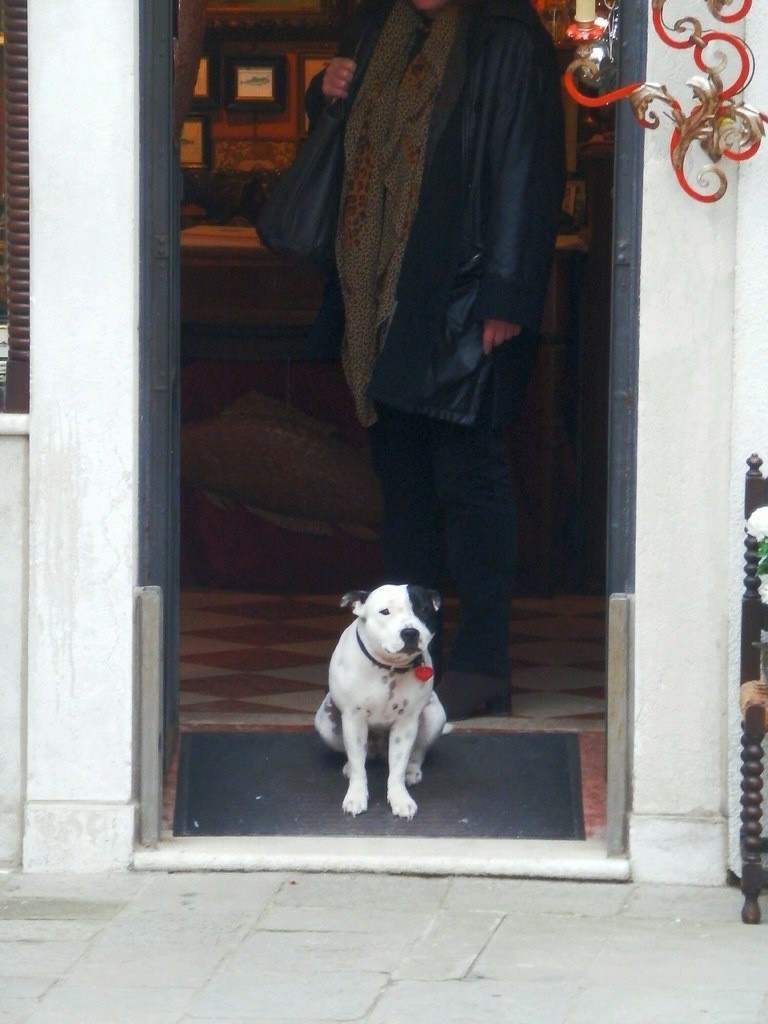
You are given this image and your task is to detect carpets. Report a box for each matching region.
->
[171,726,586,840]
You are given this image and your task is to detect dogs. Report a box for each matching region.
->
[311,583,455,818]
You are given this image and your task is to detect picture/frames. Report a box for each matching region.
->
[190,48,223,111]
[221,50,286,115]
[179,112,213,173]
[294,49,336,138]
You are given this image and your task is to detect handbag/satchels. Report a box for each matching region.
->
[257,97,345,264]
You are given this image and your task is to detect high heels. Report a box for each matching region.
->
[430,668,510,723]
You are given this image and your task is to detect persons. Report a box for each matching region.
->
[304,0,568,723]
